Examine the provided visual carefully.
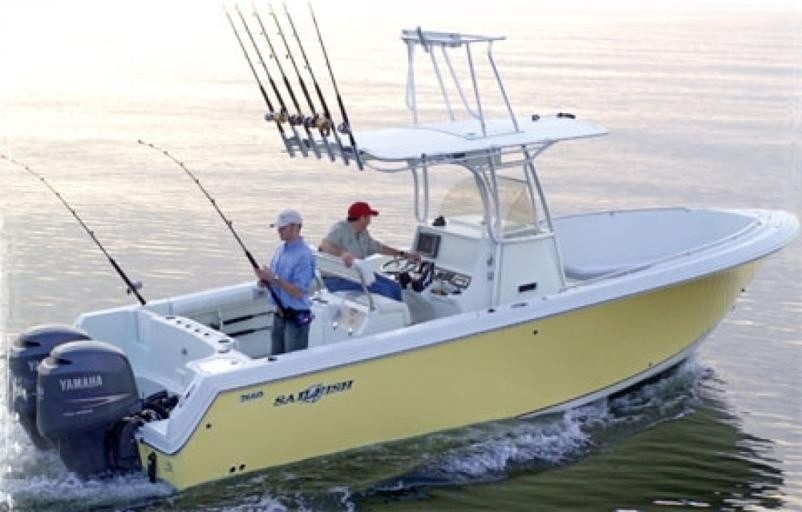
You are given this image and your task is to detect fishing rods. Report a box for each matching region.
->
[0,153,145,307]
[225,0,364,170]
[136,139,291,320]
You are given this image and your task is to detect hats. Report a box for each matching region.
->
[269,209,303,229]
[347,201,379,219]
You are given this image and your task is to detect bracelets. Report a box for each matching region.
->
[401,250,404,256]
[272,273,279,285]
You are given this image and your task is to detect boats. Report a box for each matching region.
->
[5,4,798,493]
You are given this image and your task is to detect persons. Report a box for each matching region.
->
[258,209,316,354]
[319,202,420,301]
